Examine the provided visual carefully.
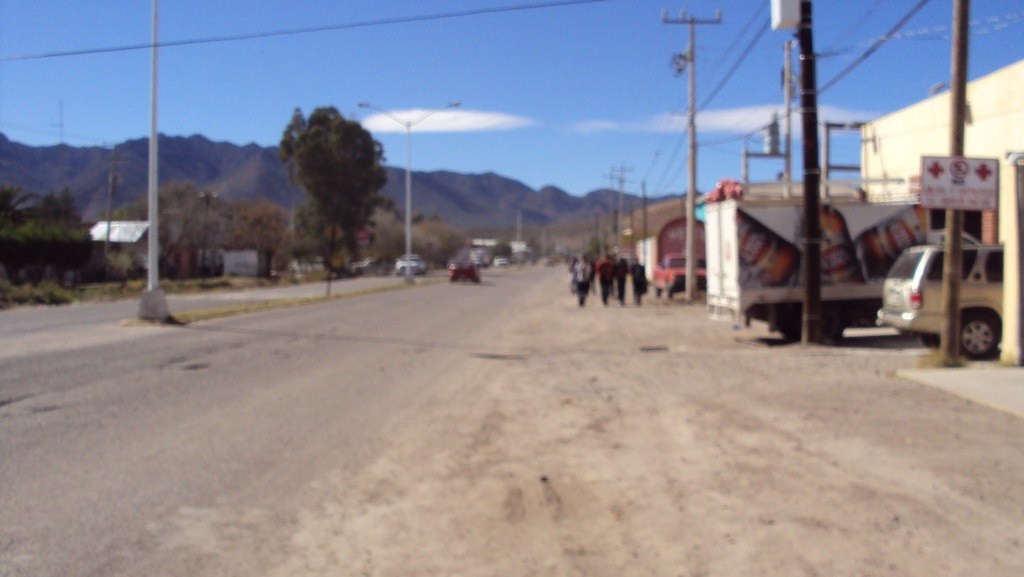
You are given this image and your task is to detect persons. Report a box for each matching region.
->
[571,253,615,307]
[631,258,645,305]
[615,259,629,303]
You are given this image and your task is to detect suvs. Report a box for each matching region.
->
[876,241,1006,361]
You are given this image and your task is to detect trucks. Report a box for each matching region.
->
[704,197,985,345]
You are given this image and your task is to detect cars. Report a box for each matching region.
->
[650,251,709,300]
[285,246,557,284]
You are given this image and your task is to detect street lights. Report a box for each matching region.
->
[356,98,464,282]
